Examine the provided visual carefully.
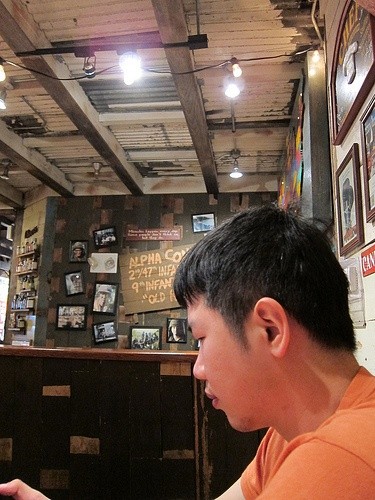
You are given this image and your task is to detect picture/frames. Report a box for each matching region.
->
[330,0,375,145]
[335,143,364,256]
[69,239,89,263]
[56,304,87,330]
[91,281,119,315]
[93,226,118,249]
[359,93,375,223]
[191,212,216,233]
[63,270,85,297]
[129,326,162,350]
[166,318,187,344]
[92,320,118,344]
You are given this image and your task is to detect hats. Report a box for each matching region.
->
[169,320,183,329]
[72,242,84,250]
[98,286,112,295]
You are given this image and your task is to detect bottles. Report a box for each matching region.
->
[14,237,38,273]
[9,274,36,335]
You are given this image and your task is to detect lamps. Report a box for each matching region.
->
[0,87,7,109]
[82,55,96,78]
[0,163,11,180]
[6,225,14,241]
[230,159,242,178]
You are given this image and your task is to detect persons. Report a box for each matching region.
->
[95,231,115,245]
[0,202,375,500]
[168,320,184,342]
[95,285,112,312]
[72,242,86,261]
[132,331,158,350]
[96,325,107,338]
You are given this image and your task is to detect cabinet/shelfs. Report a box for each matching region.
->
[8,251,40,331]
